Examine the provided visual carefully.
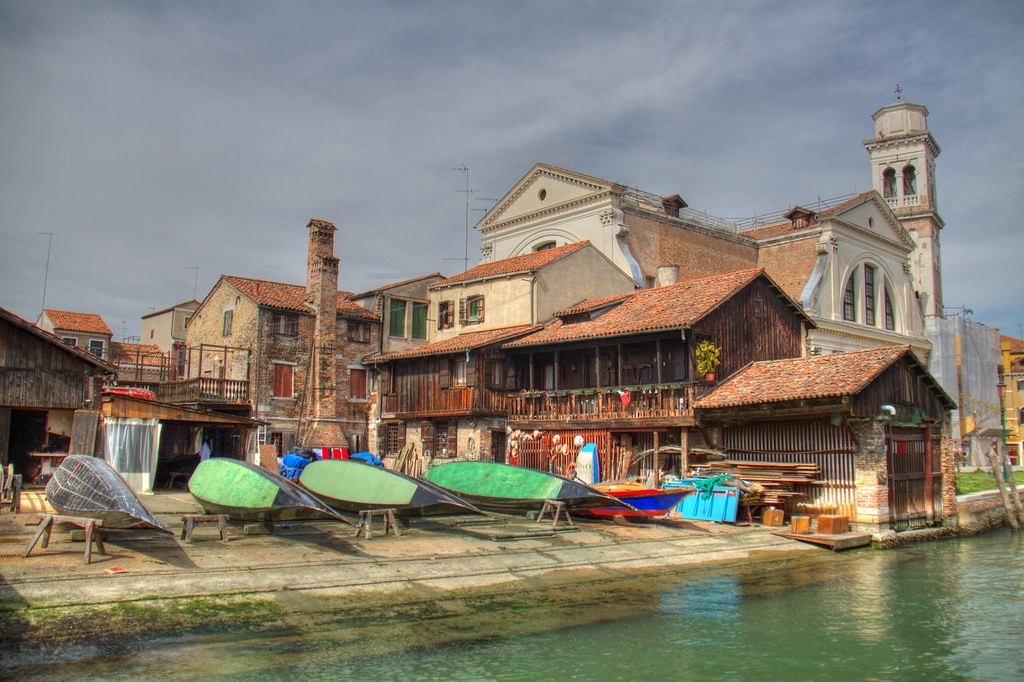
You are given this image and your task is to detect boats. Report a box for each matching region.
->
[420,464,645,521]
[45,451,176,537]
[185,458,355,530]
[567,483,697,522]
[295,458,489,518]
[593,484,649,494]
[575,442,601,484]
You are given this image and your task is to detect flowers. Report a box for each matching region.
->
[695,338,721,377]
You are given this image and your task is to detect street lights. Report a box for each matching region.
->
[38,230,53,311]
[996,362,1014,483]
[121,320,126,342]
[183,265,199,301]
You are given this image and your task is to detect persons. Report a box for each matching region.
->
[985,442,997,458]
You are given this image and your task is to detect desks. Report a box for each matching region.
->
[28,451,68,476]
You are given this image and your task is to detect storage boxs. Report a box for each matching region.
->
[761,508,784,526]
[791,516,810,535]
[816,514,849,534]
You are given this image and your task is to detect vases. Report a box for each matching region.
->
[706,373,716,381]
